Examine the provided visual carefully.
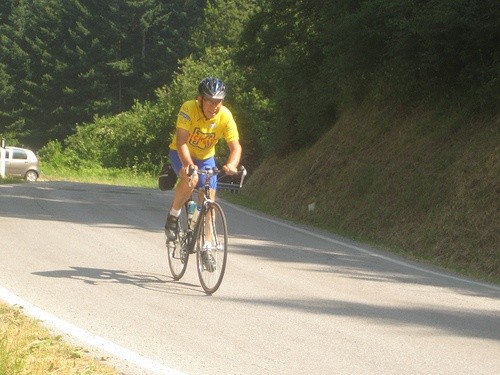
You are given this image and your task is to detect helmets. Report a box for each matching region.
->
[198,77,226,99]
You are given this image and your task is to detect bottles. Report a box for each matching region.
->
[187,200,196,219]
[190,205,202,230]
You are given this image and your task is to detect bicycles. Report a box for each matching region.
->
[164,165,248,295]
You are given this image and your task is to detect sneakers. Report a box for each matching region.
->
[201,250,216,272]
[165,211,179,240]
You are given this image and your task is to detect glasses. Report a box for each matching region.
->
[203,99,223,105]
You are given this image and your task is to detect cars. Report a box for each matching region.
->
[0,146,39,182]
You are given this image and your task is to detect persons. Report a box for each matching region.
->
[164,77,243,272]
